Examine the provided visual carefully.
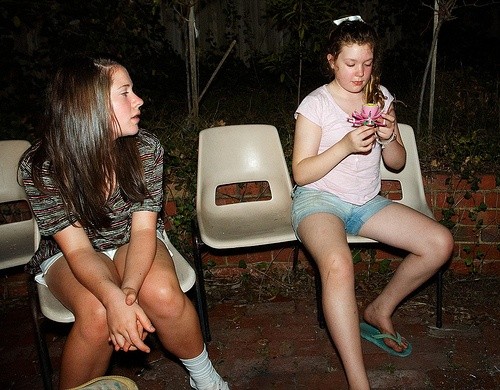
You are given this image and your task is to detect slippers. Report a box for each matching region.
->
[358,323,411,356]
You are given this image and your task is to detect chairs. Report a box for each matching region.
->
[345,124,444,328]
[34,213,197,390]
[192,122,328,343]
[0,139,52,368]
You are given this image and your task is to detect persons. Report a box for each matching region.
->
[290,16,455,390]
[18,49,229,390]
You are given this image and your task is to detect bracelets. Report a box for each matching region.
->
[375,132,398,149]
